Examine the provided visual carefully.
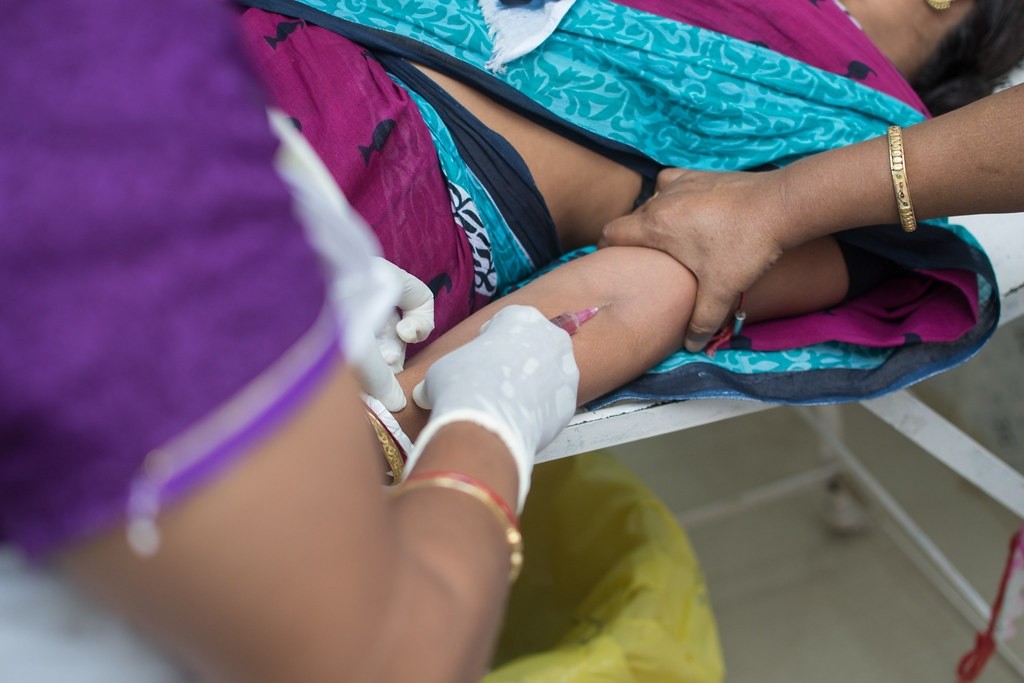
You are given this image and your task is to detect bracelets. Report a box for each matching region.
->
[364,410,405,486]
[394,471,527,586]
[887,124,919,233]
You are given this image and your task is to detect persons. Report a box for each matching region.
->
[226,0,1024,479]
[597,84,1024,352]
[0,0,581,682]
